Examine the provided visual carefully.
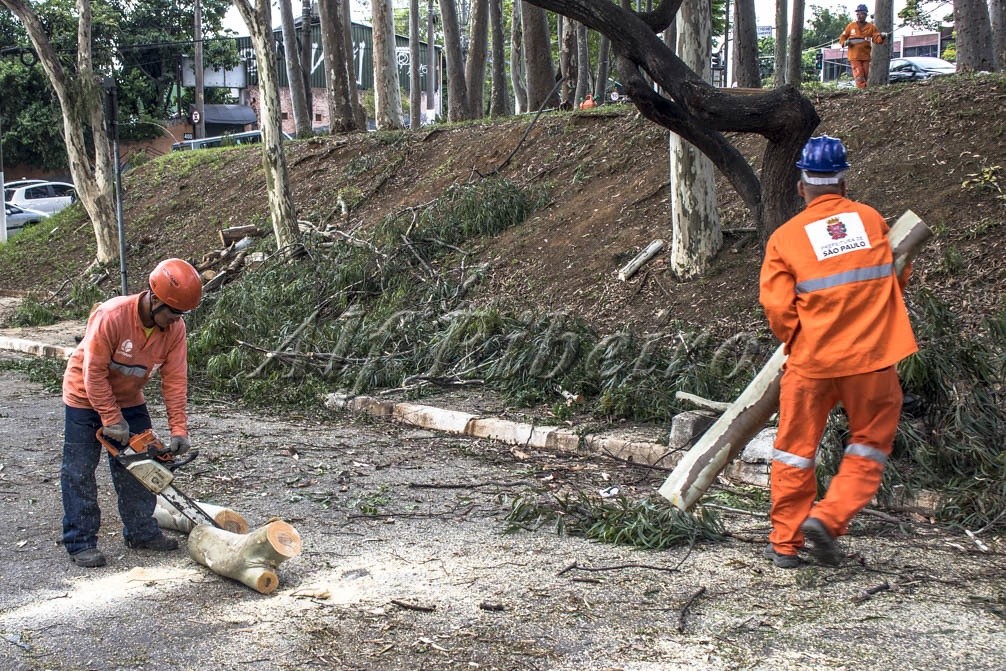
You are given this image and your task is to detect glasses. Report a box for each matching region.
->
[168,307,191,317]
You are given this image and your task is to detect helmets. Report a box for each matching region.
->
[855,4,868,12]
[795,135,851,173]
[149,258,203,311]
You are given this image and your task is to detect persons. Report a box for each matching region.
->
[60,258,202,568]
[252,135,258,144]
[839,5,884,88]
[759,135,919,567]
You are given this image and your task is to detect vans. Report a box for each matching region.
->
[172,128,293,150]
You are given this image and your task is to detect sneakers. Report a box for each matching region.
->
[803,518,838,563]
[71,548,107,567]
[763,542,800,568]
[124,531,178,551]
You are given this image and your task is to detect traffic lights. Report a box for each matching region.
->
[815,53,822,69]
[712,53,721,67]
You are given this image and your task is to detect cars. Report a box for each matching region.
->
[4,178,80,237]
[888,56,956,83]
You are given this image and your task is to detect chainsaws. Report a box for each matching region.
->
[96,425,223,529]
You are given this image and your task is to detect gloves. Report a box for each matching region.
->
[170,436,190,455]
[103,419,129,446]
[881,32,886,40]
[844,40,850,46]
